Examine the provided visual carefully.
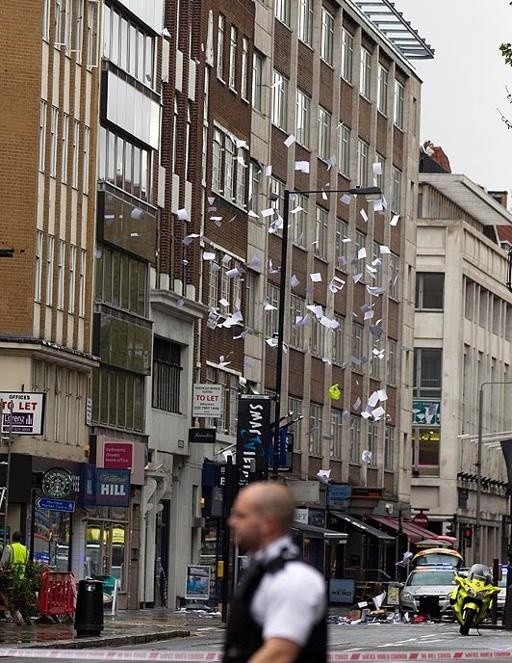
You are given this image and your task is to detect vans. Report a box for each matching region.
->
[345,567,390,582]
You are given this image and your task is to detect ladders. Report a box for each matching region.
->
[0,399,13,547]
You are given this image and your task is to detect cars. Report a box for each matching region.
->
[397,567,460,622]
[489,567,508,613]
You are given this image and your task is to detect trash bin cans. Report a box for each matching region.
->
[74,579,104,636]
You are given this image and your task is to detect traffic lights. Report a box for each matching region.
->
[464,528,471,538]
[445,519,454,536]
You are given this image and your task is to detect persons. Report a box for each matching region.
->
[223,481,329,663]
[1,531,30,591]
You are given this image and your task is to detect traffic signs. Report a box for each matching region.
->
[36,497,75,512]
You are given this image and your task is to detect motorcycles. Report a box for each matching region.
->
[449,564,503,635]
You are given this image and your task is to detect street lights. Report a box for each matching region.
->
[386,504,404,582]
